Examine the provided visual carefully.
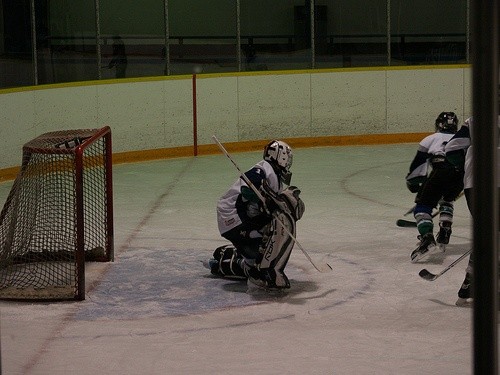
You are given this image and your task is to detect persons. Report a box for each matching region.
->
[405,112,464,264]
[445,84,500,308]
[200,140,305,295]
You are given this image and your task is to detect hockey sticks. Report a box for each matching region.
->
[210,134,334,274]
[418,247,472,281]
[396,192,465,229]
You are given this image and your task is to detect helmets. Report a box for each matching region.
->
[435,111,459,134]
[263,140,292,173]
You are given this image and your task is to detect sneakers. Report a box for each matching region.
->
[410,233,437,263]
[454,280,473,307]
[247,278,289,300]
[435,228,452,253]
[204,253,215,268]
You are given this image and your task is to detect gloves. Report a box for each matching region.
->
[406,182,421,193]
